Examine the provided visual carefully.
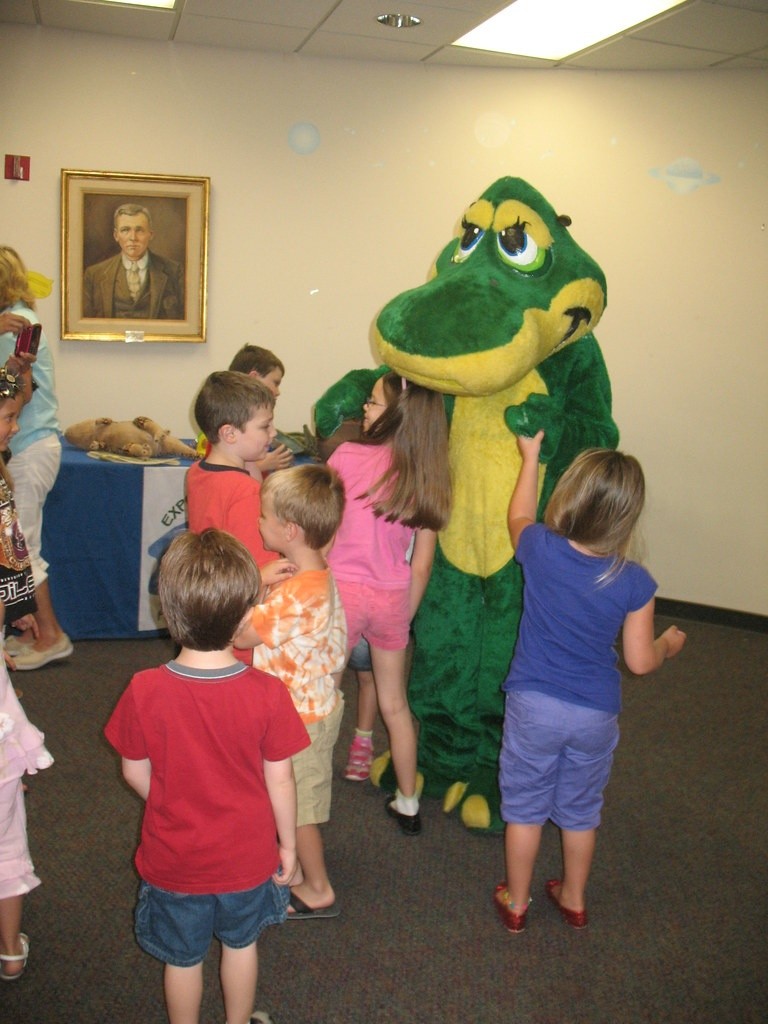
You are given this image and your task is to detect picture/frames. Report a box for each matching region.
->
[59,168,210,342]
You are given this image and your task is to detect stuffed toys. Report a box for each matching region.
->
[311,175,621,838]
[64,416,200,461]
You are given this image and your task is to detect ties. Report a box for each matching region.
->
[127,261,141,303]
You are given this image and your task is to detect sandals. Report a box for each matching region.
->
[0,933,32,981]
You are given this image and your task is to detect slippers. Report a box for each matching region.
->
[286,885,341,920]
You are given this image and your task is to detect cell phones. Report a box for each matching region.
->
[14,323,42,358]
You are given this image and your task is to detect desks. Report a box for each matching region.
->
[41,438,322,640]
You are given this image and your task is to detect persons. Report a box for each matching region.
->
[103,528,311,1024]
[494,429,687,933]
[184,341,454,921]
[0,245,75,982]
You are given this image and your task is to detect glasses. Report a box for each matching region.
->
[366,397,388,408]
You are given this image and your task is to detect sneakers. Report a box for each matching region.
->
[344,736,375,781]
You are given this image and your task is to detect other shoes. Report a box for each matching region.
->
[249,1010,275,1024]
[544,880,589,930]
[493,882,527,934]
[385,795,421,834]
[5,632,74,671]
